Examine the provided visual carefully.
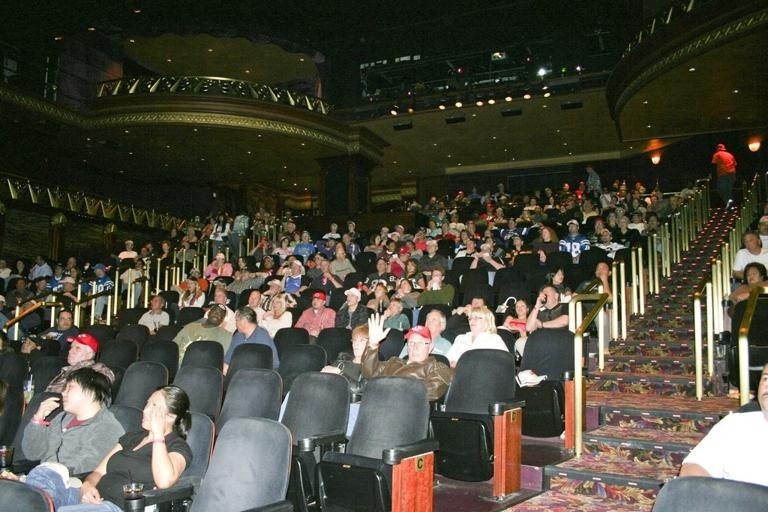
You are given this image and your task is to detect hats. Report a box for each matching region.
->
[57,221,435,339]
[67,334,99,353]
[23,333,42,346]
[566,219,578,227]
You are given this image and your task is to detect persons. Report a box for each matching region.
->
[1,180,701,512]
[678,198,766,486]
[584,162,601,190]
[709,142,738,209]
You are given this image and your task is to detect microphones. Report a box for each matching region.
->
[404,200,407,210]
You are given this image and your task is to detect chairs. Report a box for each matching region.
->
[127,408,299,512]
[519,327,591,457]
[121,412,218,512]
[726,294,767,390]
[282,372,352,509]
[437,239,455,257]
[434,348,526,501]
[81,253,614,418]
[301,375,438,510]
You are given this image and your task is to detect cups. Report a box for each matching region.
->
[23,379,36,403]
[122,482,145,498]
[0,445,14,467]
